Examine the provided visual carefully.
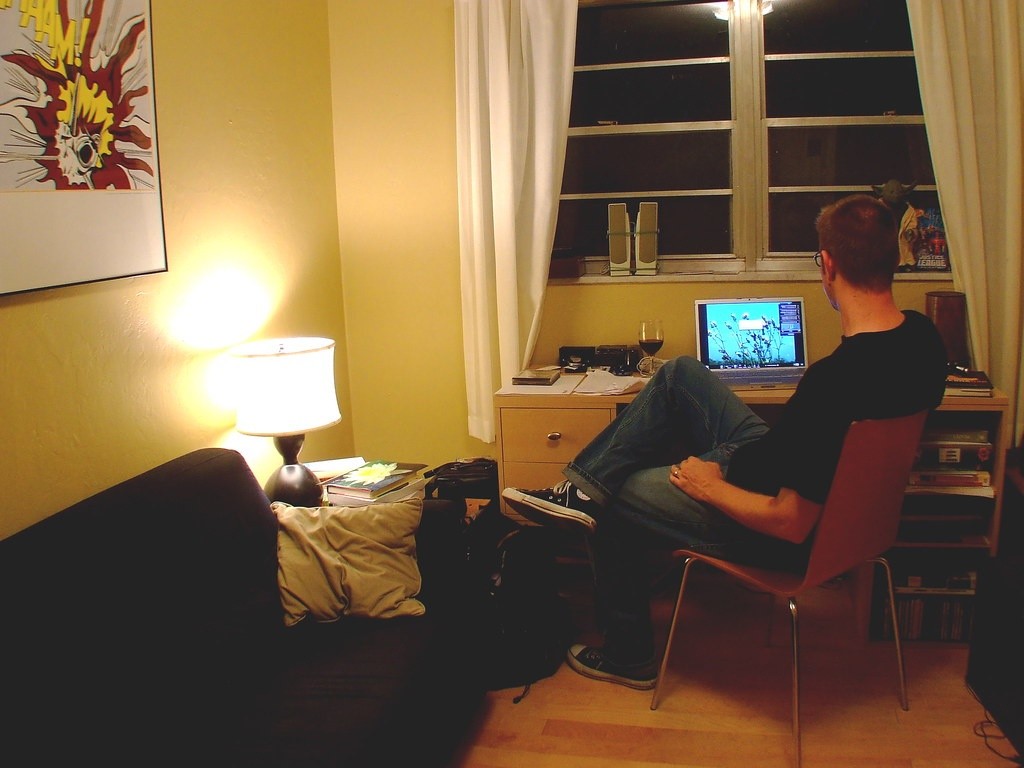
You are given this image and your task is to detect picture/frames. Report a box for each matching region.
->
[0,0,169,298]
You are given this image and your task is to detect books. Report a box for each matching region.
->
[511,369,560,386]
[941,370,1009,405]
[941,598,974,642]
[880,597,925,640]
[326,460,431,507]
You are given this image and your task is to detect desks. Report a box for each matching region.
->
[486,387,1010,642]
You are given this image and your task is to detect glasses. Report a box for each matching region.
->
[814,251,823,267]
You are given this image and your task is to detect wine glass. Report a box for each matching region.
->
[639,320,665,379]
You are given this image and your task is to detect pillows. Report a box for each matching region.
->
[268,498,427,626]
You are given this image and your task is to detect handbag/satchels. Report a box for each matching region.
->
[422,458,498,501]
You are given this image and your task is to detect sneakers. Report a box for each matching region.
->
[567,643,656,690]
[502,479,600,538]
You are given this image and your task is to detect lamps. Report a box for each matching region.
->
[228,337,343,507]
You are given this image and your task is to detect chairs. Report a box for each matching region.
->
[652,408,929,768]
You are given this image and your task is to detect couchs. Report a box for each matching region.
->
[0,449,486,768]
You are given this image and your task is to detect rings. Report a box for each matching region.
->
[674,468,679,476]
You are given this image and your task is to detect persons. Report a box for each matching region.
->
[501,195,948,688]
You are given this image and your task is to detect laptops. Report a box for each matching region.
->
[695,296,808,391]
